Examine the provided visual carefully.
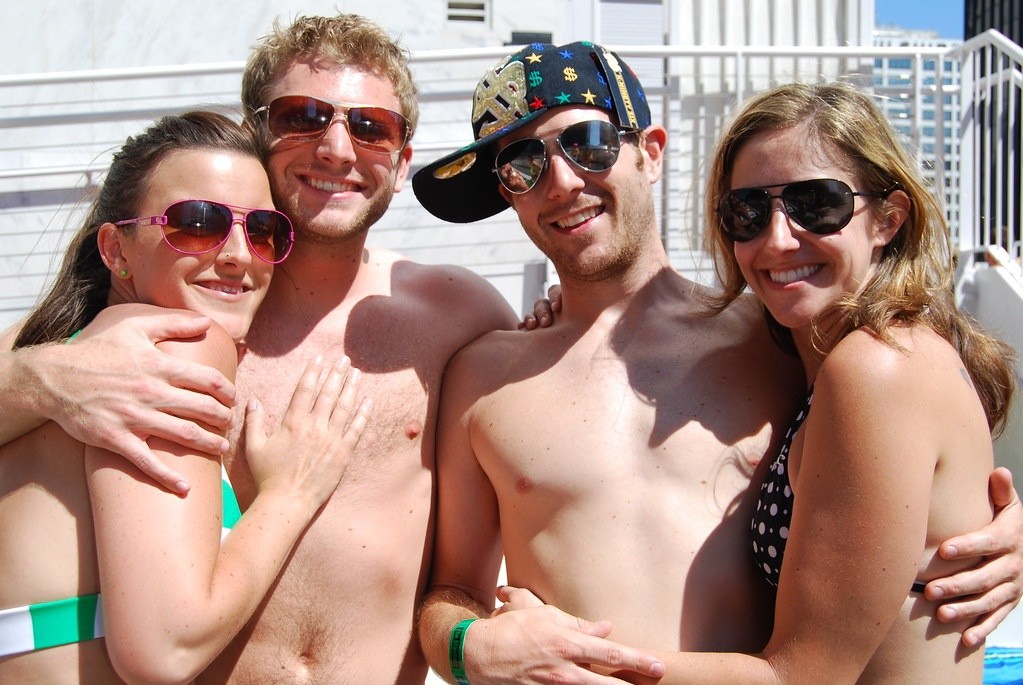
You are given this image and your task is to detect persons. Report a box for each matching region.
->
[0,111,374,685]
[525,154,542,182]
[411,40,1023,685]
[491,83,1018,685]
[0,14,522,685]
[570,143,579,157]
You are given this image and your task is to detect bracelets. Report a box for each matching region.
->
[448,618,477,685]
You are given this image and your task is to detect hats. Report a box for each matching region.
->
[412,40,651,223]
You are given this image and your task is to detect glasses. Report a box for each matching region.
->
[253,94,413,155]
[491,119,643,195]
[115,200,295,264]
[716,179,899,243]
